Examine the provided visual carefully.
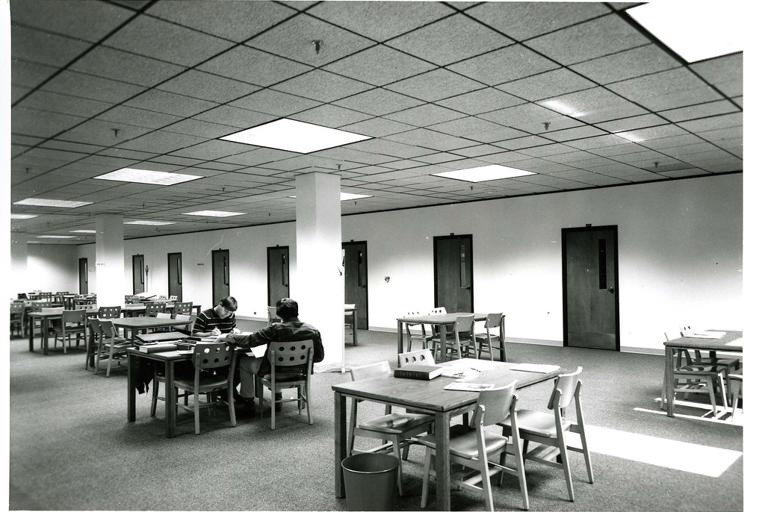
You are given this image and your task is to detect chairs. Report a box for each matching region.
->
[660,326,741,417]
[403,307,502,362]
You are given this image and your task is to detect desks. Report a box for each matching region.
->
[395,314,507,369]
[328,358,562,510]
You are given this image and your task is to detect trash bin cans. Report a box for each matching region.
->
[341,452,399,509]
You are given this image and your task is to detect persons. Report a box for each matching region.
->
[192,295,243,406]
[212,298,325,416]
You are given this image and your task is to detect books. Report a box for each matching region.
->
[440,364,489,379]
[206,335,220,339]
[200,338,213,342]
[139,343,179,354]
[250,343,269,358]
[511,362,561,374]
[443,381,496,394]
[393,363,444,380]
